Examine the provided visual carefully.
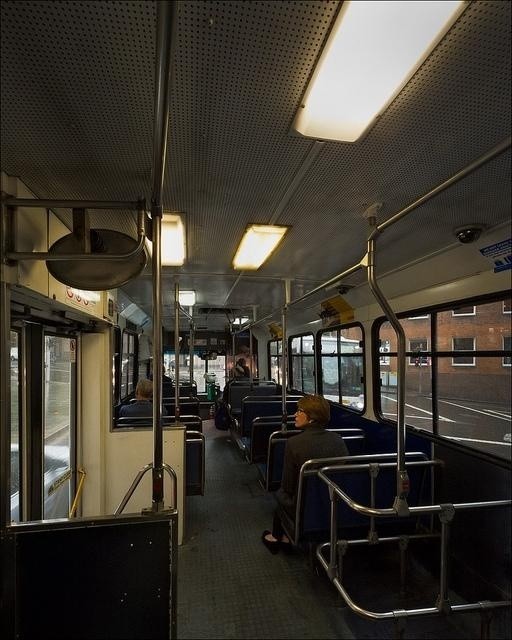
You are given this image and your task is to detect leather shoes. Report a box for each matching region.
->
[262,530,292,554]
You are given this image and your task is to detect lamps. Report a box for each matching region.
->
[175,290,197,309]
[232,223,287,272]
[142,215,184,268]
[292,1,467,143]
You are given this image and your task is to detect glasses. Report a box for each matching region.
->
[297,409,305,415]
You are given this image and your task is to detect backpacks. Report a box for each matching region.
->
[215,402,231,431]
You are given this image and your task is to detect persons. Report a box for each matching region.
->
[223,365,248,407]
[148,364,173,397]
[261,393,350,554]
[234,357,251,379]
[117,377,170,424]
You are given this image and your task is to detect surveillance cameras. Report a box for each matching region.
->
[456,224,485,244]
